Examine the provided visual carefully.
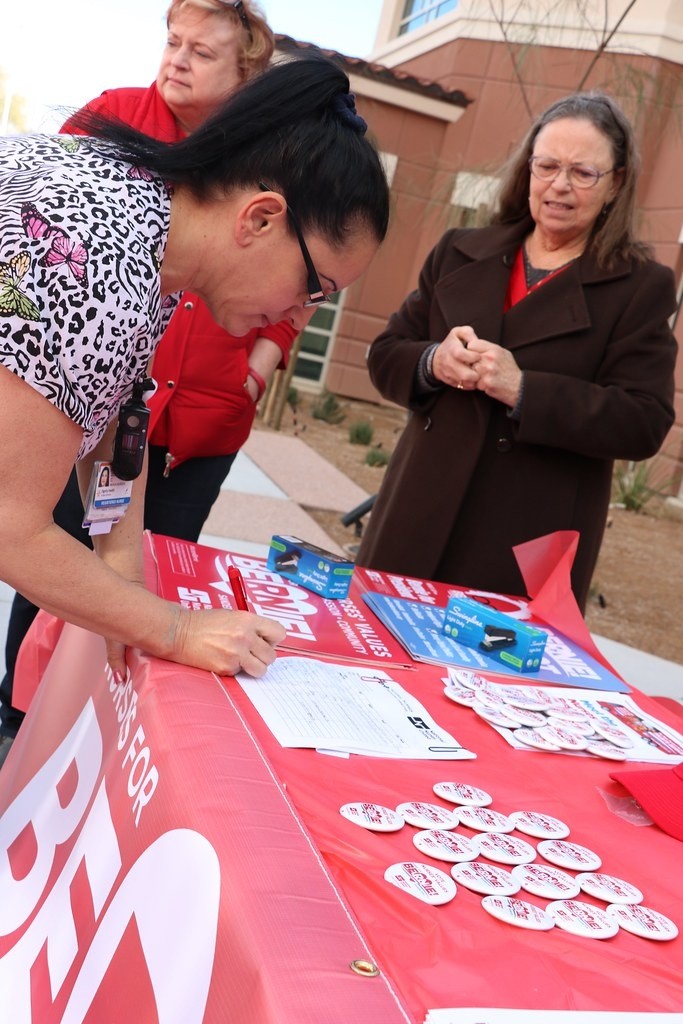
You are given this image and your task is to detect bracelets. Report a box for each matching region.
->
[249,368,266,401]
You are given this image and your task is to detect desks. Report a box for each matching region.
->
[118,529,683,1024]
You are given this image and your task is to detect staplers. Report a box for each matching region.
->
[274,550,302,575]
[479,624,518,653]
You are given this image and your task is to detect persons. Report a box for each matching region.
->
[0,56,391,677]
[356,96,678,619]
[0,0,299,768]
[99,467,109,487]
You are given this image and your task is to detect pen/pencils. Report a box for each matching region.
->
[225,565,251,611]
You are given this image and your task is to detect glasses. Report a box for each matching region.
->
[527,157,616,189]
[219,0,253,43]
[258,181,331,307]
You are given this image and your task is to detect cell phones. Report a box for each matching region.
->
[112,404,151,480]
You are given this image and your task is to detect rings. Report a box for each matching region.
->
[457,381,463,389]
